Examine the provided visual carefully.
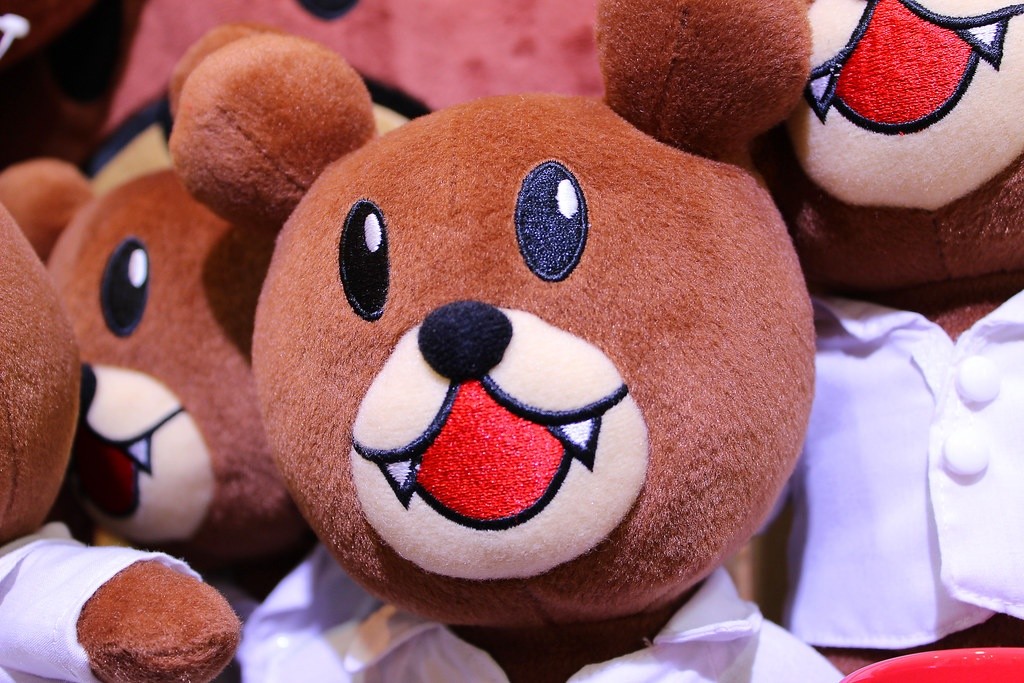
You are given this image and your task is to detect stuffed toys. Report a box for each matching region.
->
[1,1,1024,683]
